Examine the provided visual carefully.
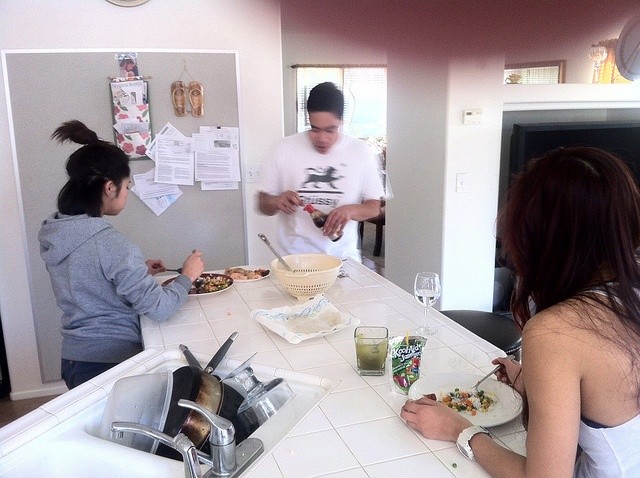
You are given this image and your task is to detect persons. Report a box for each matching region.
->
[399,146,640,478]
[255,81,384,267]
[37,117,206,393]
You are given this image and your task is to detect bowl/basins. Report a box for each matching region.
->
[100,370,174,454]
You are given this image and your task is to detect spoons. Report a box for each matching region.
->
[192,248,204,288]
[257,233,294,271]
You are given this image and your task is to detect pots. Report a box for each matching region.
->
[178,344,260,447]
[155,332,259,460]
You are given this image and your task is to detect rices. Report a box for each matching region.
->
[469,395,481,409]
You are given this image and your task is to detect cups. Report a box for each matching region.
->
[355,326,389,376]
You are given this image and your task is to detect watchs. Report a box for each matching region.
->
[456,420,491,464]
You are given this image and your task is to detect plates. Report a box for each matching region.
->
[251,294,358,345]
[408,372,525,429]
[161,272,234,304]
[224,266,271,288]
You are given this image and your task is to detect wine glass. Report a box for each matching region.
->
[413,273,441,340]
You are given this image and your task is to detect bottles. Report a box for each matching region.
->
[303,203,343,242]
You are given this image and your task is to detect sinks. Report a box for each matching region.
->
[83,359,295,464]
